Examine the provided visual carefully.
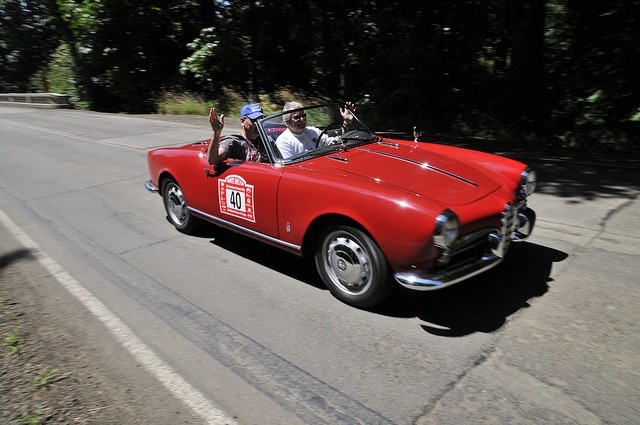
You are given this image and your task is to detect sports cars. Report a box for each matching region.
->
[145,99,540,305]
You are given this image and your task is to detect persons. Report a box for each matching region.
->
[205,103,283,166]
[277,102,357,159]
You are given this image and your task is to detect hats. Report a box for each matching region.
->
[238,103,267,119]
